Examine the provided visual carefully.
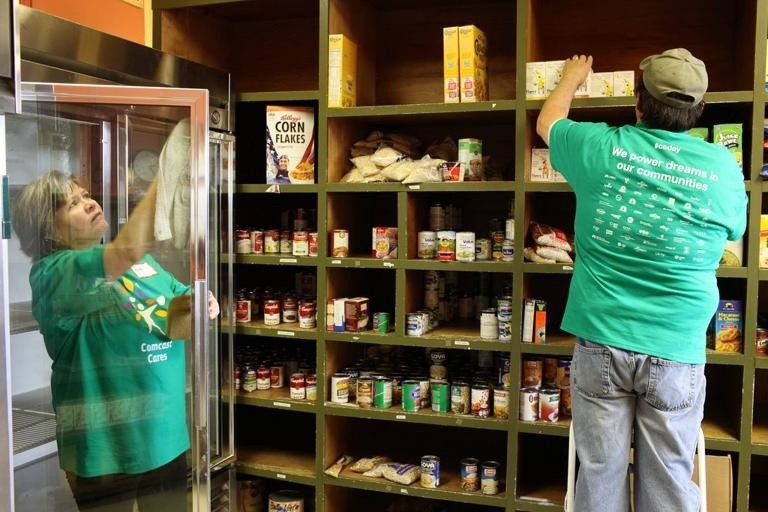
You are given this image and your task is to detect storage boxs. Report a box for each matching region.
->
[628,448,734,512]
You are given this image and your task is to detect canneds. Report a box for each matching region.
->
[305,375,317,400]
[471,385,490,418]
[488,230,504,250]
[279,230,292,254]
[373,312,390,333]
[356,376,374,408]
[445,272,460,296]
[499,321,512,341]
[523,356,546,388]
[545,355,558,383]
[251,230,263,253]
[480,307,498,325]
[264,230,279,253]
[501,240,514,261]
[400,379,420,411]
[478,461,501,496]
[499,354,511,387]
[477,350,493,368]
[330,228,350,258]
[496,298,512,322]
[242,366,257,392]
[388,372,406,403]
[270,364,284,388]
[255,364,271,390]
[451,382,470,414]
[236,297,252,323]
[444,204,458,231]
[456,232,478,261]
[262,299,281,325]
[374,378,392,408]
[538,389,561,422]
[288,373,305,400]
[430,350,448,380]
[406,313,423,337]
[429,202,445,231]
[342,367,360,400]
[415,376,430,407]
[476,237,492,260]
[331,374,349,403]
[457,290,474,318]
[426,289,440,308]
[560,384,572,417]
[459,458,478,492]
[417,230,435,259]
[308,232,317,257]
[473,296,489,319]
[520,387,539,421]
[478,325,498,339]
[233,367,242,389]
[555,357,574,388]
[234,229,251,253]
[490,251,501,259]
[493,388,509,419]
[298,301,315,328]
[423,269,438,289]
[249,292,259,314]
[430,382,450,412]
[420,455,441,489]
[435,230,457,262]
[281,297,298,323]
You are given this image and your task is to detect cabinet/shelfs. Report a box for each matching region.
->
[153,1,768,512]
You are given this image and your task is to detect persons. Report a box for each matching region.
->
[13,118,223,512]
[535,48,748,511]
[273,155,292,183]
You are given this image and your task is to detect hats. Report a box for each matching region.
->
[639,47,709,109]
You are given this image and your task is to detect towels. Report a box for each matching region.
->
[154,118,191,249]
